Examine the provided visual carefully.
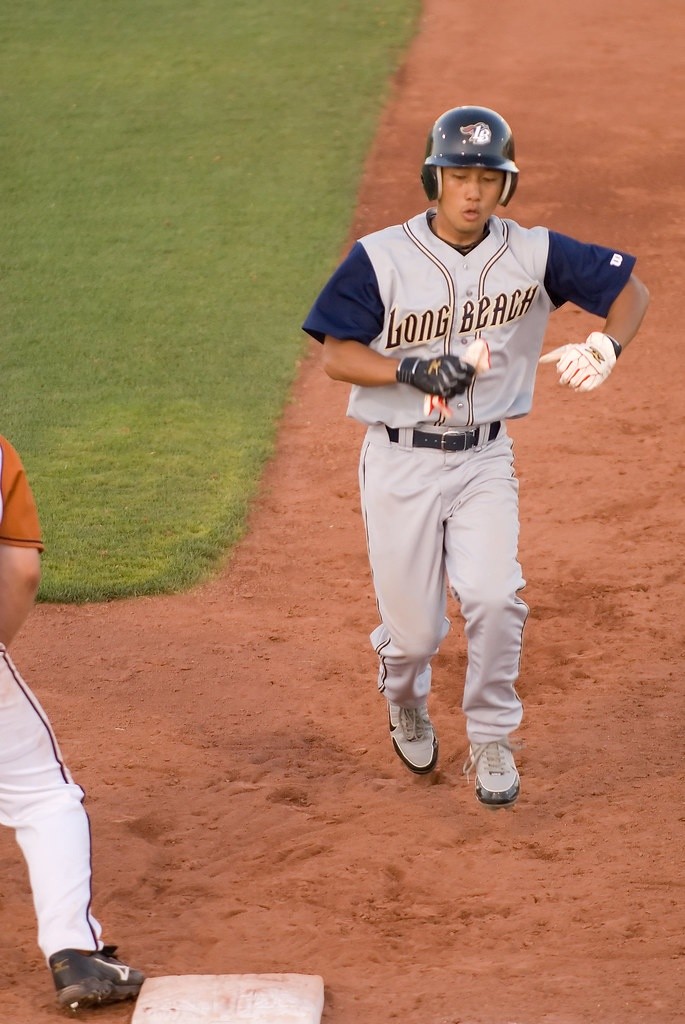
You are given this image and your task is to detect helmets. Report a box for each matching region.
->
[420,106,520,208]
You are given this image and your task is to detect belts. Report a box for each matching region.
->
[382,420,502,450]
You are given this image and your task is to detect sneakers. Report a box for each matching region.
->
[385,698,444,774]
[463,742,521,809]
[49,943,145,1015]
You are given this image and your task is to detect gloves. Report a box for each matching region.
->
[539,331,622,393]
[396,352,476,401]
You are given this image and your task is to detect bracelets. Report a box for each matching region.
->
[605,332,622,360]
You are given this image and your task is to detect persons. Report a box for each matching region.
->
[0,432,145,1008]
[303,106,652,804]
